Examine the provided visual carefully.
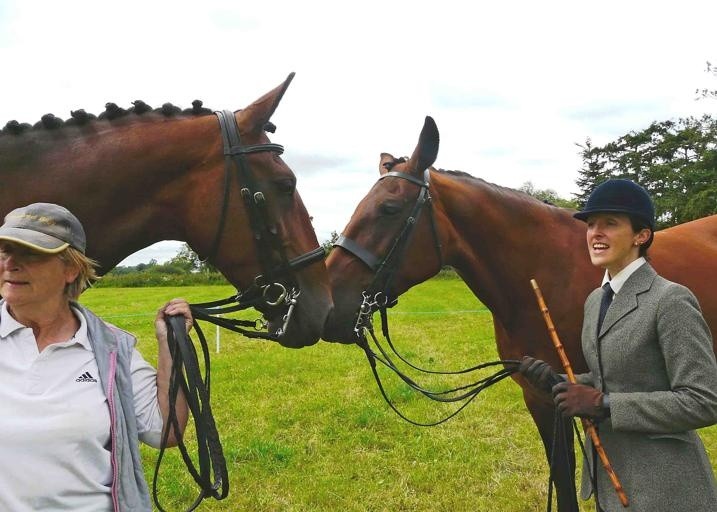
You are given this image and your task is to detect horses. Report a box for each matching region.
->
[320,114,717,512]
[0,72,336,350]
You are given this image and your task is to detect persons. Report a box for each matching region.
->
[519,179,717,512]
[1,202,193,512]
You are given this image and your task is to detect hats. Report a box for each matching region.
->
[1,203,86,253]
[573,180,654,229]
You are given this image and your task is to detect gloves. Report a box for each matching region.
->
[551,383,605,420]
[519,356,564,392]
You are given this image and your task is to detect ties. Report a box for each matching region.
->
[597,283,614,336]
